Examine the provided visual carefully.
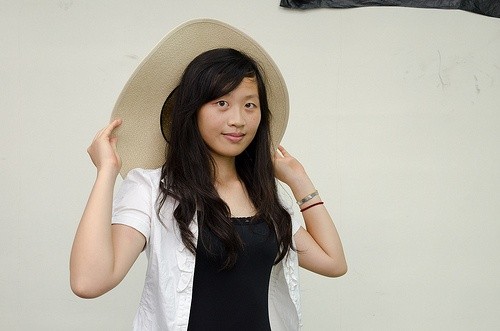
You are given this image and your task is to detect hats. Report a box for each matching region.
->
[109,18,289,179]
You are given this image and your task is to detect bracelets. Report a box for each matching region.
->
[296,190,319,206]
[300,202,325,212]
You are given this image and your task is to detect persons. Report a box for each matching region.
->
[69,48,348,331]
